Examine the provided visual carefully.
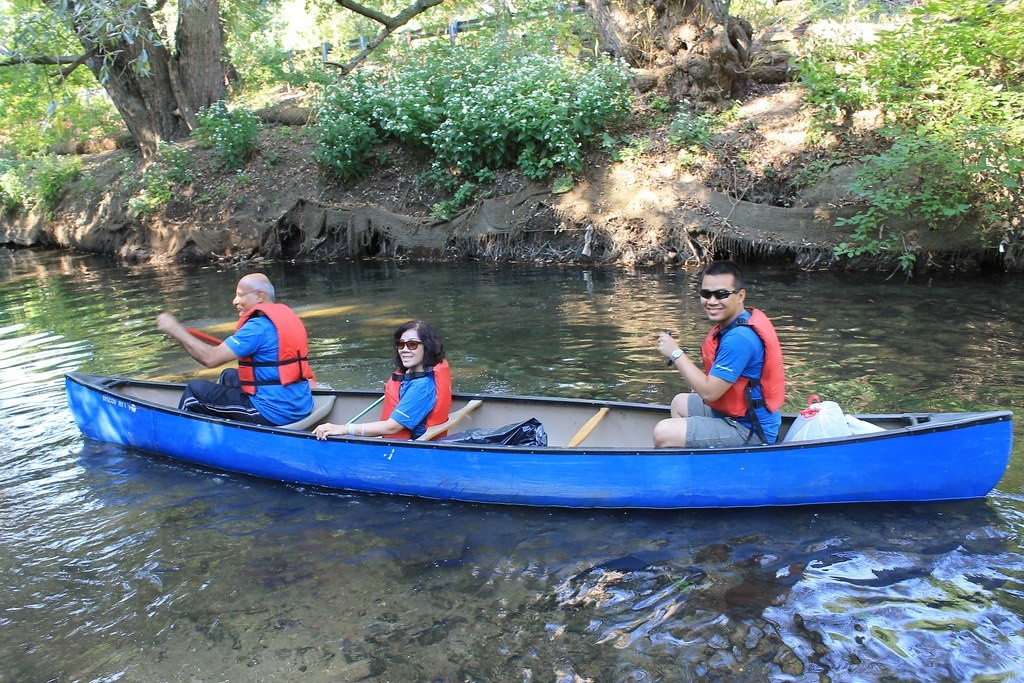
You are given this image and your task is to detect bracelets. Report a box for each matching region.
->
[361,422,365,437]
[348,423,354,436]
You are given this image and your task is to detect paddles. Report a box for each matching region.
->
[186,326,222,344]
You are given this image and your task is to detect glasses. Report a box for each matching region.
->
[394,339,423,350]
[699,288,738,300]
[235,290,259,298]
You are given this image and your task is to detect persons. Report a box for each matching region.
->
[310,318,453,442]
[652,260,787,449]
[156,271,316,427]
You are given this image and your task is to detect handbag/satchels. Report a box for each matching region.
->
[784,393,853,443]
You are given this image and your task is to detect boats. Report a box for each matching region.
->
[63,370,1015,508]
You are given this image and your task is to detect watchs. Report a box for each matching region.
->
[667,349,684,367]
